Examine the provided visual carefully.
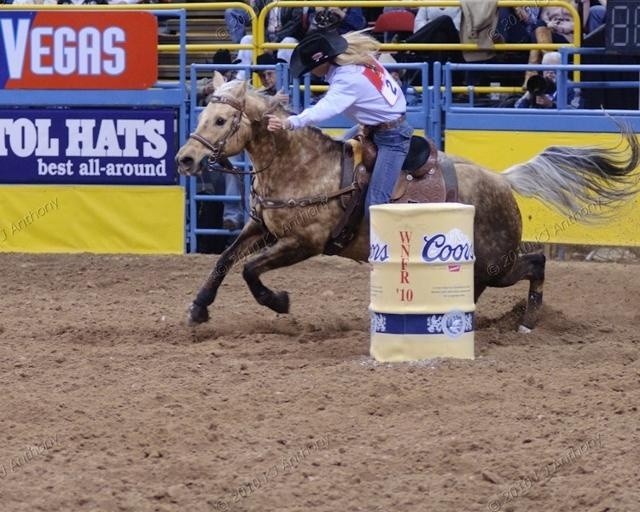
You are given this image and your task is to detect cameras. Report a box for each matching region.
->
[527,76,557,98]
[314,8,339,28]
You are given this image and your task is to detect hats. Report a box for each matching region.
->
[257,53,289,66]
[210,50,241,65]
[526,74,555,97]
[289,33,347,78]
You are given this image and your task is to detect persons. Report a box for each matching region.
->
[185,47,246,254]
[265,30,415,263]
[183,1,608,114]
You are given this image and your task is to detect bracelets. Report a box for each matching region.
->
[282,121,286,134]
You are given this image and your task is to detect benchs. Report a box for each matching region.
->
[150,0,251,81]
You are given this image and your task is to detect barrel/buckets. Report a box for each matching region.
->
[368,202,476,361]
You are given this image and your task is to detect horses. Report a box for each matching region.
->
[174,68,640,336]
